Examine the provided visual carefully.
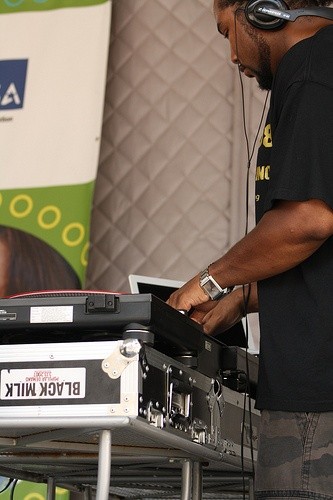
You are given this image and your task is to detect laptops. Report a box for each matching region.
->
[129,274,261,355]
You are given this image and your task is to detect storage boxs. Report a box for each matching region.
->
[1,338,259,463]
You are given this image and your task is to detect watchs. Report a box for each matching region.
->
[200,263,234,300]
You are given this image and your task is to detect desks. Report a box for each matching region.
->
[0,417,255,500]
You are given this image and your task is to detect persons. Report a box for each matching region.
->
[165,1,333,500]
[1,225,83,296]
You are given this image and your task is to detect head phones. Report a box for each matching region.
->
[245,0,333,31]
